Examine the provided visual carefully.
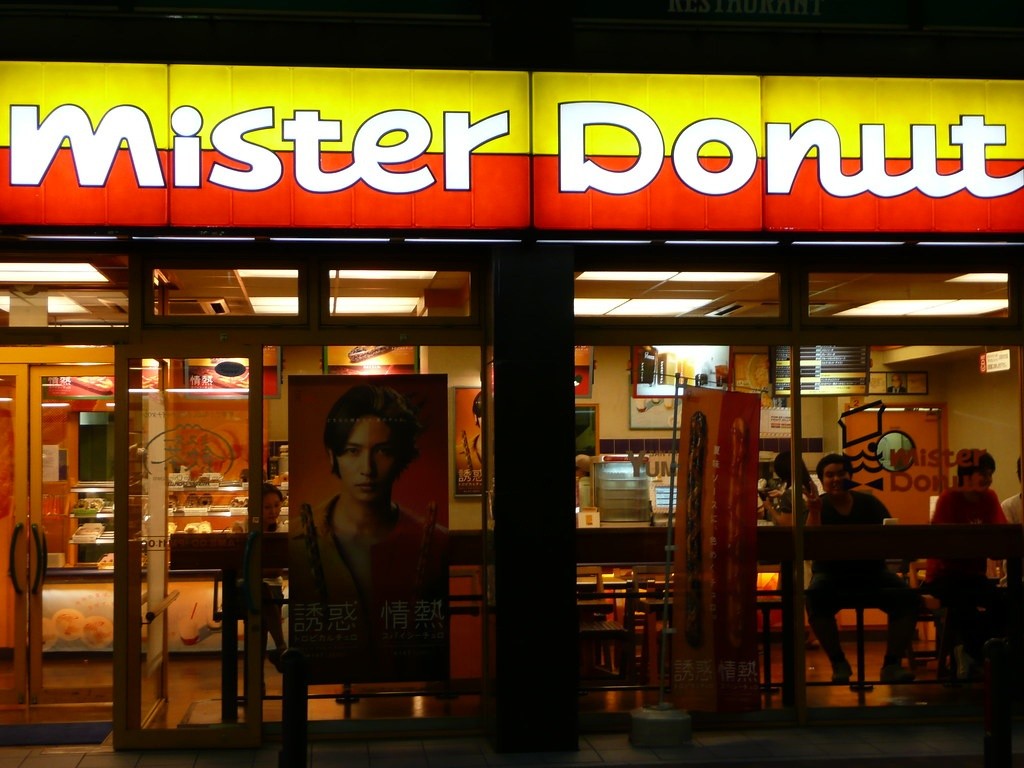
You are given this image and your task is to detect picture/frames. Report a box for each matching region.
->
[868,370,929,396]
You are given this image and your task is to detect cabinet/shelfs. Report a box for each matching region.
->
[67,482,289,544]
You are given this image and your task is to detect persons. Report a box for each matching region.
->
[764,446,1024,688]
[455,391,483,493]
[227,483,286,673]
[888,374,906,393]
[290,384,448,681]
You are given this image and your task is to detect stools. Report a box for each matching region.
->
[756,600,783,693]
[642,599,675,687]
[848,605,877,692]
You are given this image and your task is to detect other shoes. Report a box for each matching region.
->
[831,657,853,685]
[276,641,287,655]
[880,666,916,682]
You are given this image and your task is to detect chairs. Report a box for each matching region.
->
[906,561,949,684]
[576,565,627,672]
